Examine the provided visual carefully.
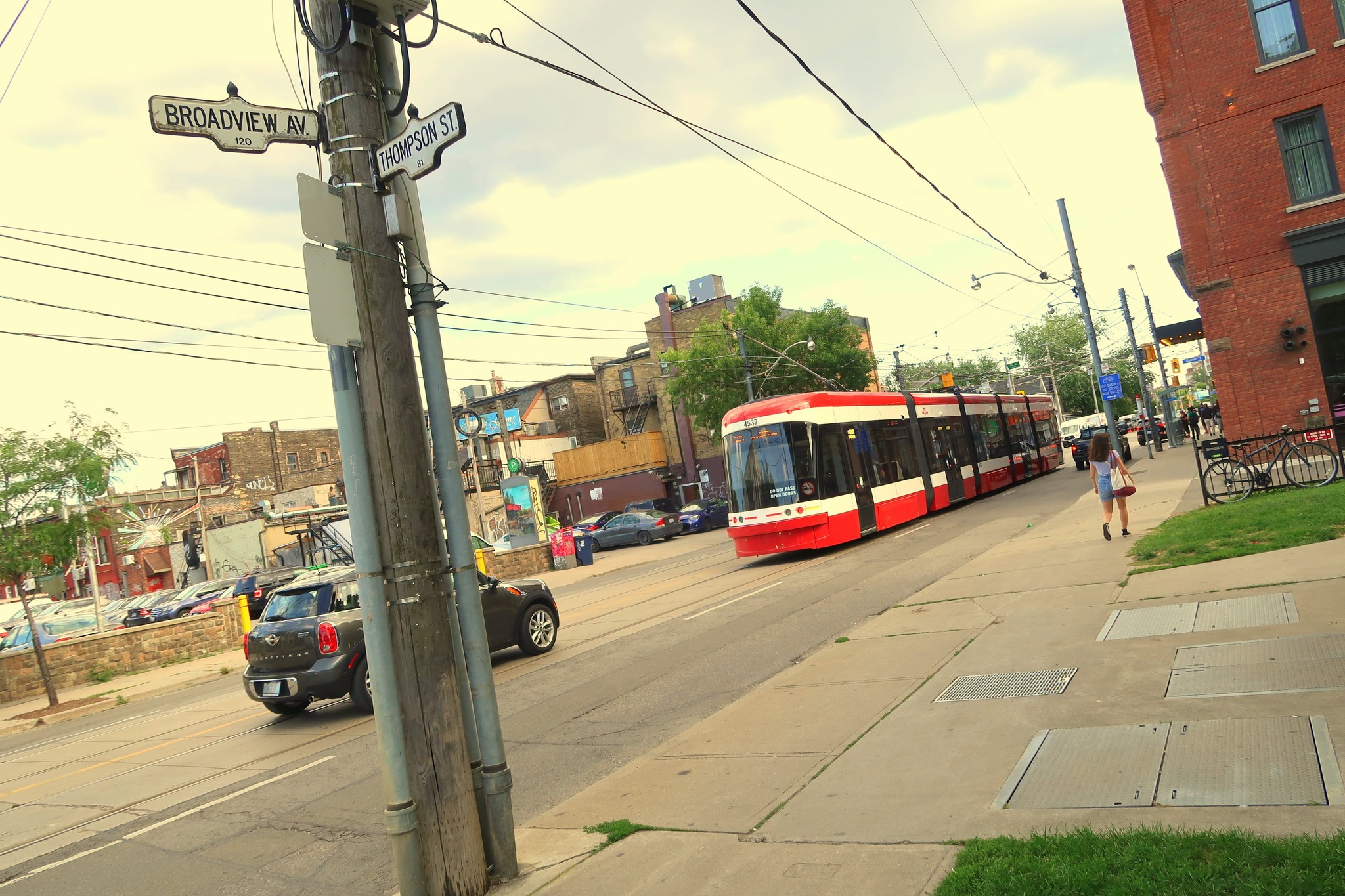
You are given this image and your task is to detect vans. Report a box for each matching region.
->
[624,498,685,513]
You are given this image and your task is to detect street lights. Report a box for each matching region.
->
[1010,356,1048,395]
[174,451,213,580]
[1093,370,1117,413]
[746,336,817,401]
[1047,303,1163,452]
[971,273,1123,466]
[1054,365,1085,424]
[899,352,951,390]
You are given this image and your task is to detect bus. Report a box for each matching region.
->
[721,391,1064,559]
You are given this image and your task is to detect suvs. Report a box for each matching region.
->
[441,519,509,564]
[243,557,559,716]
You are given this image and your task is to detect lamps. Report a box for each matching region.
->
[1227,97,1233,105]
[696,464,700,468]
[592,480,595,484]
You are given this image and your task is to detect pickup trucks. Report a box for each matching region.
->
[992,441,1035,458]
[1072,423,1131,471]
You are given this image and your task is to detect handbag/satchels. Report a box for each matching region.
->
[1109,450,1136,497]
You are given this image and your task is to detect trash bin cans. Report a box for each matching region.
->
[550,527,577,571]
[545,516,561,540]
[573,535,594,567]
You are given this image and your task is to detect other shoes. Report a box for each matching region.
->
[1102,522,1111,541]
[1191,437,1196,441]
[1185,435,1190,438]
[1197,437,1199,440]
[1219,434,1223,438]
[1122,532,1131,538]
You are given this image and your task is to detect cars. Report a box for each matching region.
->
[0,567,310,655]
[678,497,729,532]
[491,525,585,550]
[569,510,623,534]
[1116,421,1127,435]
[584,509,683,553]
[1137,414,1169,446]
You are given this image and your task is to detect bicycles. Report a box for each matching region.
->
[1202,425,1339,505]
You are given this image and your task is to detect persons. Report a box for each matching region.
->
[1131,420,1138,430]
[1087,432,1135,541]
[862,450,915,489]
[1179,410,1190,438]
[1198,400,1224,438]
[1187,406,1200,440]
[328,487,335,504]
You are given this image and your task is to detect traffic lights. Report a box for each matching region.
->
[1138,347,1152,361]
[1192,396,1195,401]
[1016,391,1025,396]
[1170,359,1181,374]
[1212,380,1215,385]
[1187,404,1190,408]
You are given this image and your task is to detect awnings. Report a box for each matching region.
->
[143,552,171,575]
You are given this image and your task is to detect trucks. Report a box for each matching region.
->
[1118,408,1148,422]
[1060,412,1108,448]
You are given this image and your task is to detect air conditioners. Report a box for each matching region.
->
[122,555,135,566]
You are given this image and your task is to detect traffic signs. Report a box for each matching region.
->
[1099,374,1123,401]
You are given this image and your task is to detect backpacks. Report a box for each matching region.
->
[1189,412,1197,425]
